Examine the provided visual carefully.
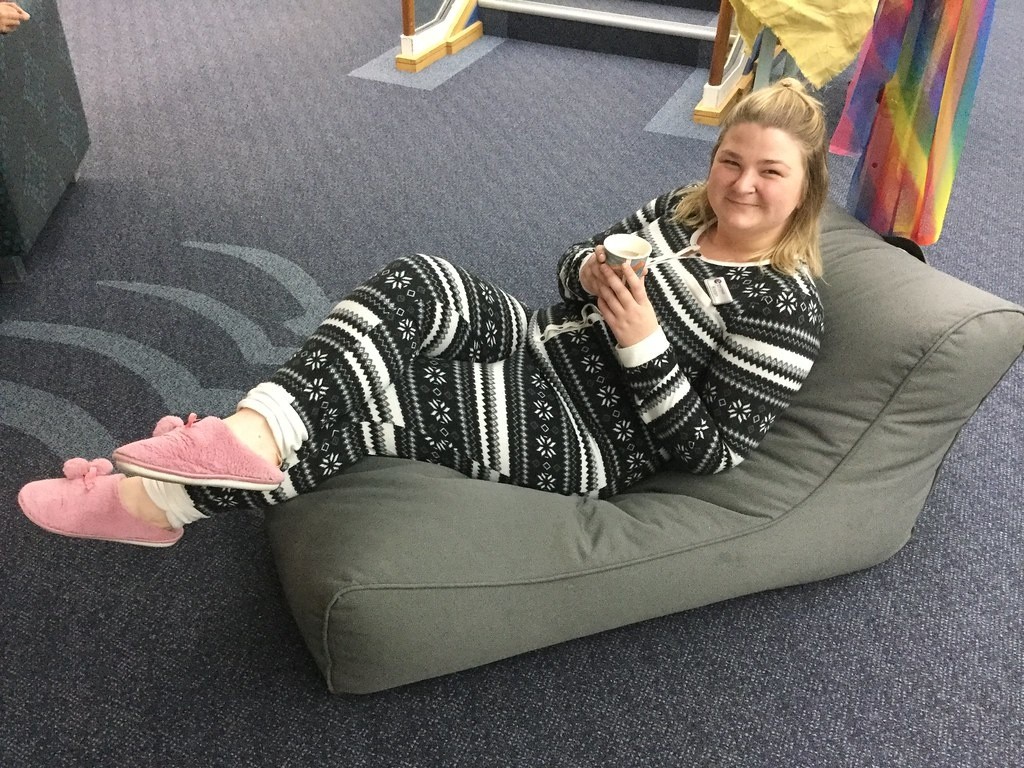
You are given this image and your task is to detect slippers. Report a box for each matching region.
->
[17,458,184,547]
[113,412,285,491]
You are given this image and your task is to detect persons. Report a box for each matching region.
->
[0,0,31,33]
[16,76,831,549]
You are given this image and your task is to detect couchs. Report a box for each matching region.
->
[262,192,1023,695]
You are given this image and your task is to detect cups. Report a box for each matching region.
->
[604,233,652,292]
